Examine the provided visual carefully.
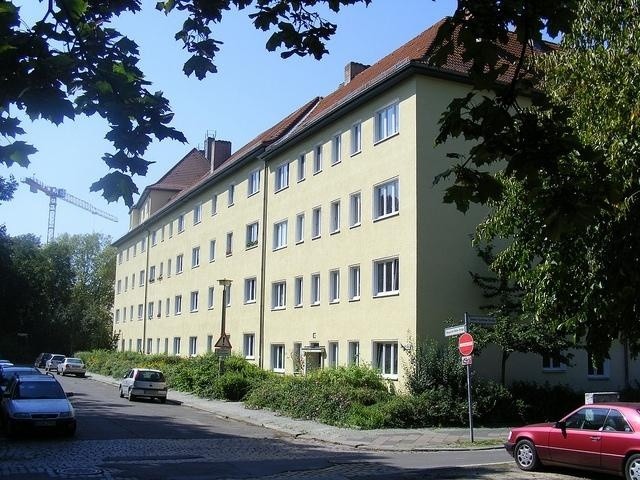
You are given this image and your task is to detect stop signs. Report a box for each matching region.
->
[458,333,475,356]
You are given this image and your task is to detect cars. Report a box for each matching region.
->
[34,351,87,377]
[0,358,77,437]
[117,366,168,403]
[503,401,640,479]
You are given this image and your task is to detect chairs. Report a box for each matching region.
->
[607,418,620,430]
[140,374,158,380]
